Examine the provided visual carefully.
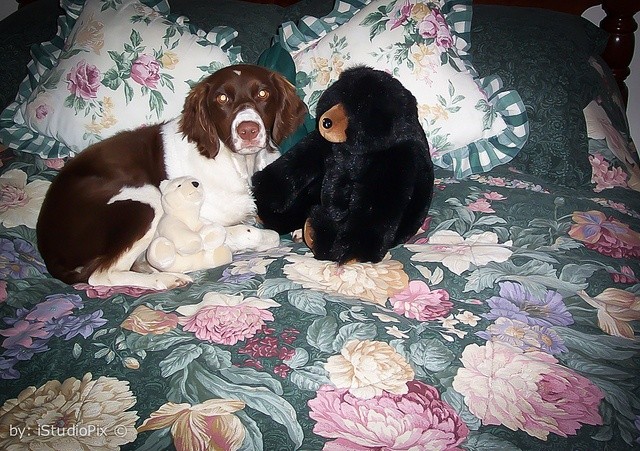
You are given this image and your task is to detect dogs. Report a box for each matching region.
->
[38,66,308,291]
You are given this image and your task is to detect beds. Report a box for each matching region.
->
[0,1,640,450]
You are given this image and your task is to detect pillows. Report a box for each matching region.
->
[1,0,310,111]
[0,3,243,160]
[252,0,531,182]
[276,0,592,187]
